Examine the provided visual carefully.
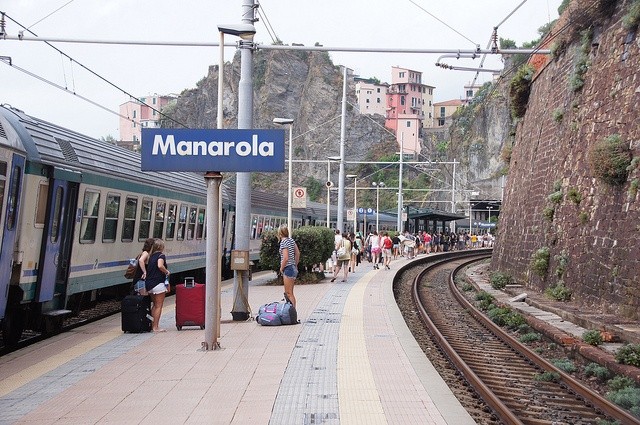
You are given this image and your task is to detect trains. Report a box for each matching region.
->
[0,99,500,361]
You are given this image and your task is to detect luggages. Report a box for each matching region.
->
[121,291,152,334]
[175,276,205,330]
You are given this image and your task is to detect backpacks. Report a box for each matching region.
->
[384,236,392,249]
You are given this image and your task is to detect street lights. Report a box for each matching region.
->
[328,156,343,276]
[198,24,259,349]
[371,180,386,235]
[346,173,357,234]
[273,116,306,294]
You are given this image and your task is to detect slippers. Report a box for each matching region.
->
[153,328,166,332]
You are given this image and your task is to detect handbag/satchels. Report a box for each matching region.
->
[124,253,141,279]
[256,302,300,325]
[336,247,345,258]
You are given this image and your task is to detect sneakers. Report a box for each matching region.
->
[341,279,347,282]
[331,277,336,282]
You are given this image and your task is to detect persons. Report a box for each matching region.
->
[133,238,155,296]
[145,239,170,331]
[277,223,300,309]
[313,228,495,283]
